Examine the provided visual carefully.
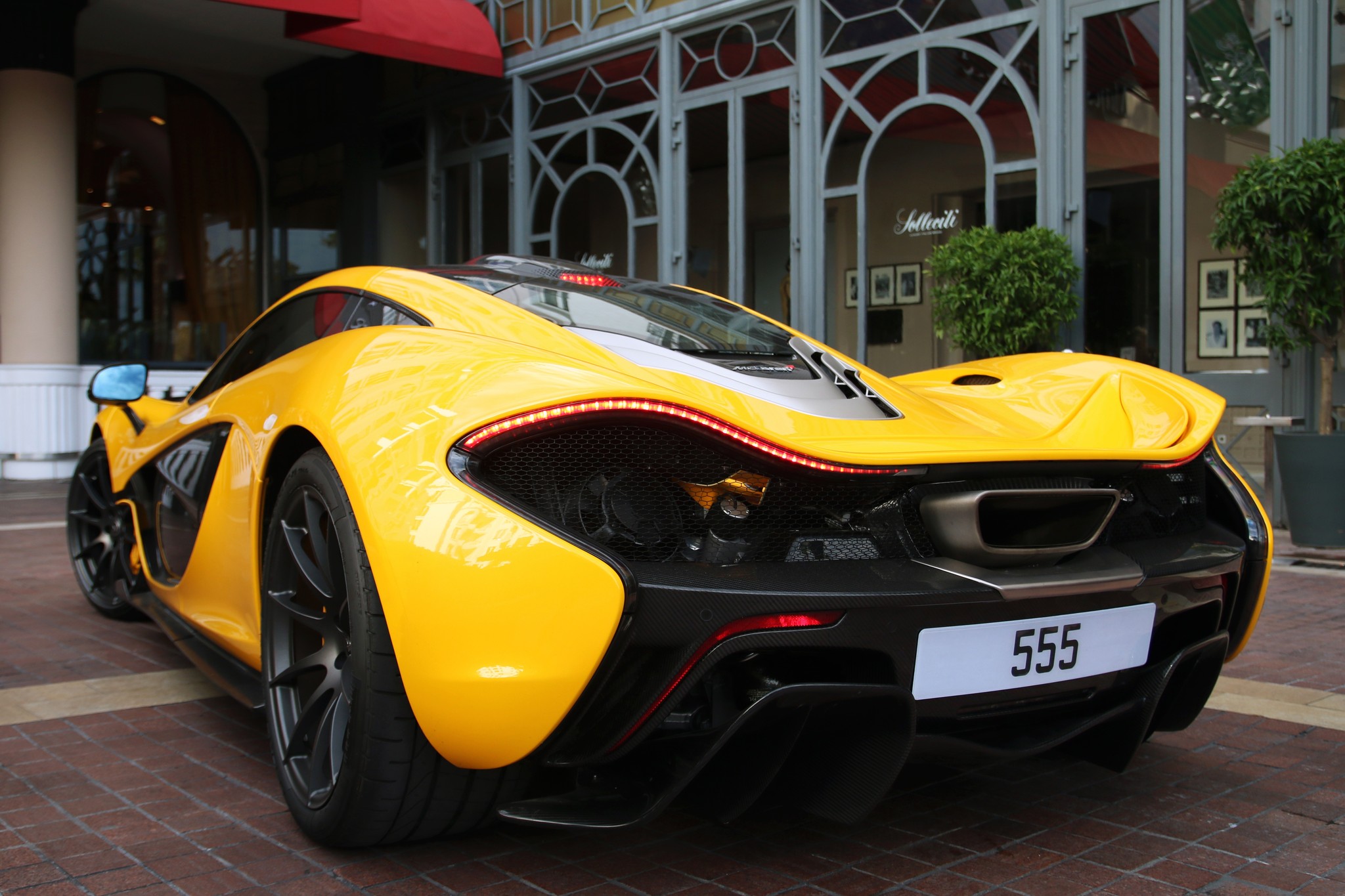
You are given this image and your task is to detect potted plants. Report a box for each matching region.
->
[1214,138,1345,549]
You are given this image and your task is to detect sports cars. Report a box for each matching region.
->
[63,257,1273,861]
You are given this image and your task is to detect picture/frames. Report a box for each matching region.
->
[844,262,925,308]
[1198,256,1271,358]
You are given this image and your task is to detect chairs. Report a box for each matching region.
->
[315,292,348,336]
